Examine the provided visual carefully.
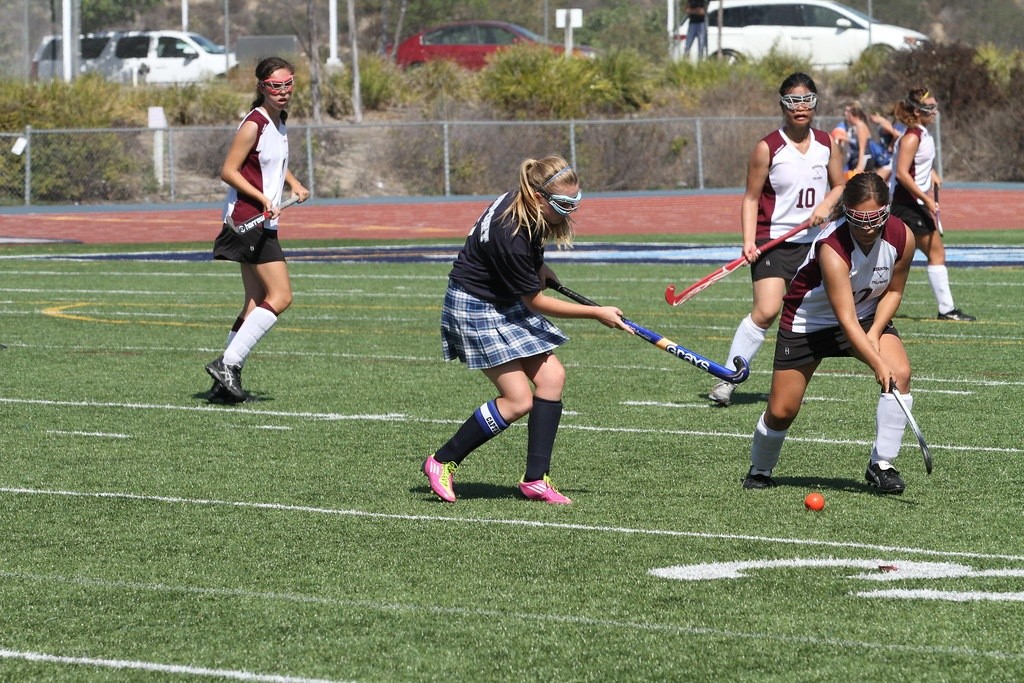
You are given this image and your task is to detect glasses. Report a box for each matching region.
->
[780,92,817,110]
[534,187,581,217]
[258,75,294,95]
[842,203,891,230]
[906,96,938,115]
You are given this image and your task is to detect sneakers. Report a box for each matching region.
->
[864,459,906,492]
[708,381,738,406]
[205,355,247,403]
[518,472,571,505]
[743,465,776,490]
[937,308,976,321]
[419,452,459,503]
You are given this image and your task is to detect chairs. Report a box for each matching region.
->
[162,38,178,57]
[483,27,496,43]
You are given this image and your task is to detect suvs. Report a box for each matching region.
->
[28,31,240,86]
[672,0,935,73]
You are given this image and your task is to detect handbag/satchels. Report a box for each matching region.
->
[868,138,890,167]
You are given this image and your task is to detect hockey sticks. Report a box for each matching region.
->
[543,274,751,383]
[888,377,934,476]
[663,203,846,305]
[224,195,301,234]
[933,181,945,235]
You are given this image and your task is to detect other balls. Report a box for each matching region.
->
[804,494,825,511]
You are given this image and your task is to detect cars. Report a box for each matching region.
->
[386,21,607,70]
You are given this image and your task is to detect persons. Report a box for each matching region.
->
[205,57,311,405]
[743,171,917,495]
[888,86,977,322]
[681,0,710,66]
[708,71,848,407]
[419,154,637,505]
[830,101,909,190]
